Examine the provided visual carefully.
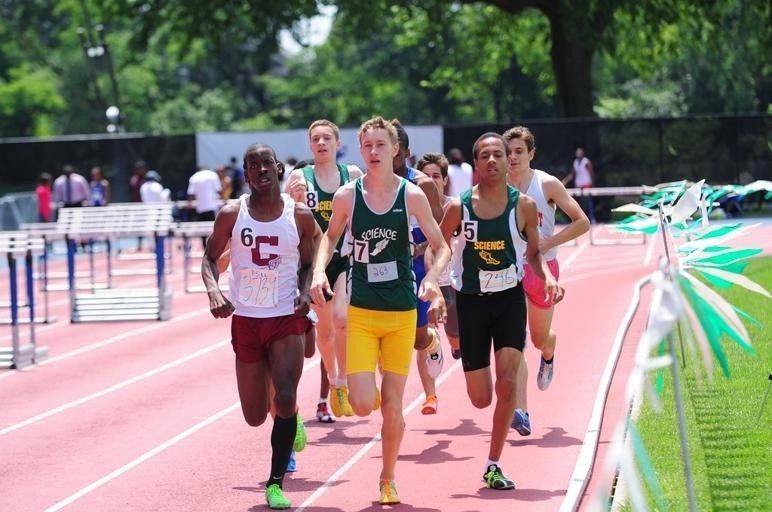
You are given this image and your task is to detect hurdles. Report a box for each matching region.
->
[174,221,232,294]
[57,203,173,325]
[0,221,111,368]
[557,185,651,247]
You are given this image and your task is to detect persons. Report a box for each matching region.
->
[217,161,324,476]
[281,113,595,480]
[199,143,317,508]
[308,117,453,508]
[34,152,316,249]
[423,131,568,493]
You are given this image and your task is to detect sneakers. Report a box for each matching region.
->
[373,387,381,410]
[377,478,401,505]
[329,384,357,418]
[420,393,439,415]
[285,411,308,473]
[264,483,292,510]
[314,401,337,423]
[445,334,462,360]
[481,463,516,490]
[535,351,555,392]
[509,406,532,437]
[424,328,445,380]
